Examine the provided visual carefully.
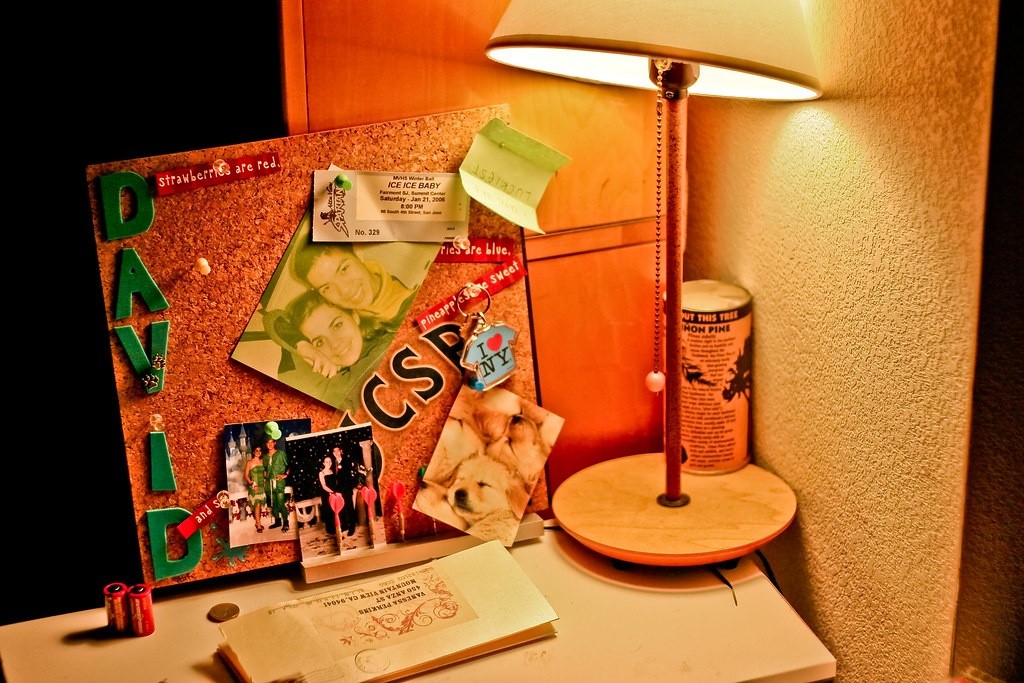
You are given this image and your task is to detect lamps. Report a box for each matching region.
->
[485,0,831,570]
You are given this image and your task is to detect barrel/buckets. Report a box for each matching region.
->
[662,279,752,476]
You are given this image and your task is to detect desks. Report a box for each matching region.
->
[0,527,840,683]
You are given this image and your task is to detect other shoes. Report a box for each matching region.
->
[281,520,289,532]
[255,524,263,530]
[269,518,281,529]
[255,524,262,533]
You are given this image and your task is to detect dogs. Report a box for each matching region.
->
[440,453,531,546]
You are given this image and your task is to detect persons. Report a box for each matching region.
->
[262,242,435,415]
[319,446,360,536]
[244,436,291,532]
